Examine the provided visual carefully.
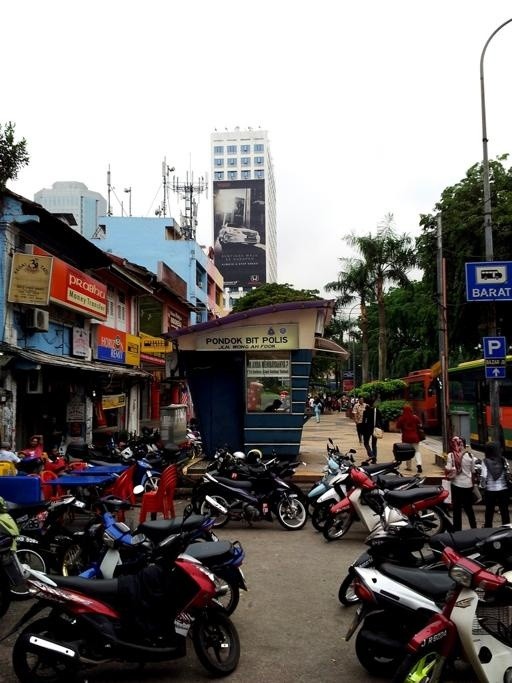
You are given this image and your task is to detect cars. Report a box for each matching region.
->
[218,223,260,245]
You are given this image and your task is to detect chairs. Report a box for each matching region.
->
[40,460,176,522]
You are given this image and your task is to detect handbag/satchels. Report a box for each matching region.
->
[442,467,457,504]
[418,428,425,441]
[472,487,483,505]
[317,403,322,408]
[372,427,384,439]
[505,473,512,490]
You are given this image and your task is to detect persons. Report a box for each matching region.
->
[351,398,370,443]
[350,393,360,409]
[0,442,21,463]
[264,399,282,413]
[480,441,512,528]
[30,437,42,454]
[309,386,338,423]
[360,399,383,464]
[396,404,423,473]
[444,435,476,531]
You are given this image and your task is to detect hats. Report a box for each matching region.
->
[1,441,11,448]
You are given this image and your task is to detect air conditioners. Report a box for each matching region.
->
[24,306,50,332]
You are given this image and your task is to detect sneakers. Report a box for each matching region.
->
[366,456,376,463]
[417,464,422,473]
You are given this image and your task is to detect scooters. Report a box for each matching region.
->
[0,428,512,681]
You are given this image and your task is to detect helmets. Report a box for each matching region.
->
[232,448,262,466]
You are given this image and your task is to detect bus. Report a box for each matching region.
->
[402,355,512,451]
